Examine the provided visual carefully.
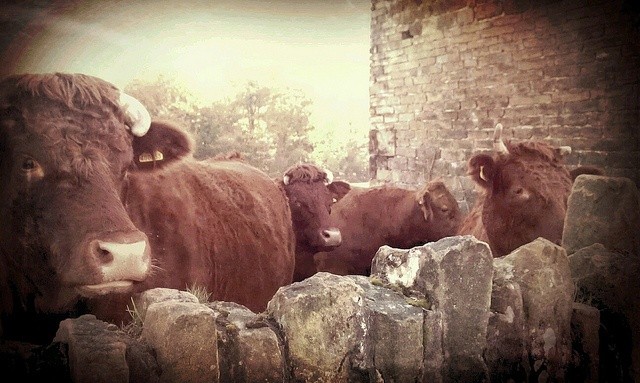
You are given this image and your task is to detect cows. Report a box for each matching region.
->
[0,71,295,343]
[313,180,465,276]
[271,160,351,283]
[454,122,606,257]
